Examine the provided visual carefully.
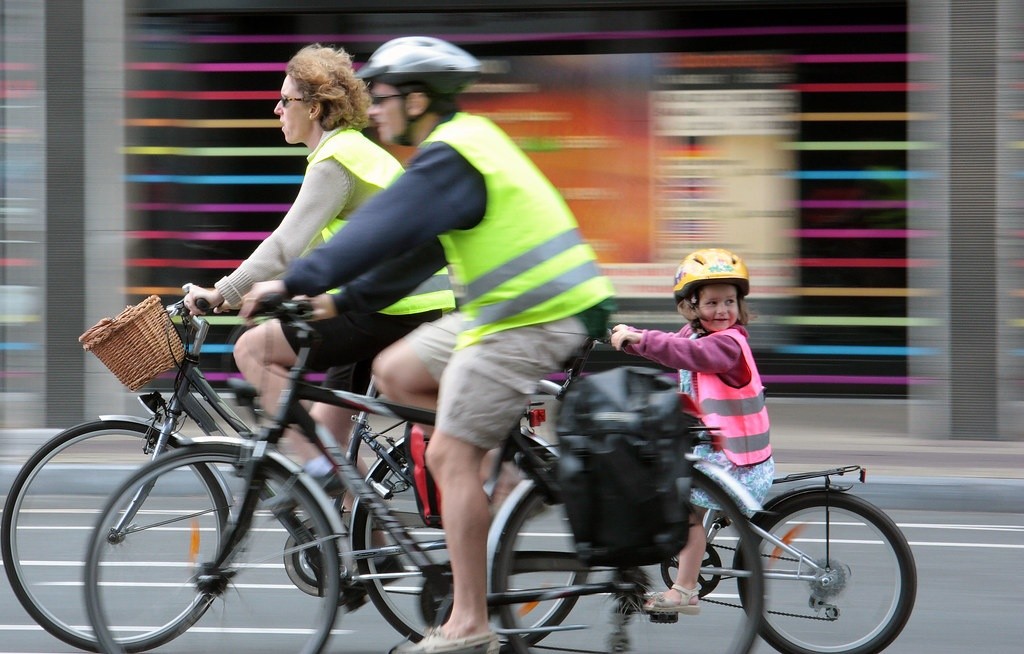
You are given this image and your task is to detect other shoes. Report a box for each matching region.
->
[402,626,499,654]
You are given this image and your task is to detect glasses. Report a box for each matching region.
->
[280,93,315,106]
[368,90,410,104]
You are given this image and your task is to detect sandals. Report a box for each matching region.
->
[647,583,703,615]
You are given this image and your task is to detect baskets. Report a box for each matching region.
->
[80,294,186,391]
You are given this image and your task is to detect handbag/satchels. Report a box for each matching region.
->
[559,368,689,565]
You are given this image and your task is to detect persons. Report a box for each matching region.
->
[184,42,456,613]
[611,248,775,616]
[238,36,620,654]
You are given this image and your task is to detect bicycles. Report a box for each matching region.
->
[1,283,918,654]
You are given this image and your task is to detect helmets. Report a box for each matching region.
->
[673,248,750,299]
[352,36,487,96]
